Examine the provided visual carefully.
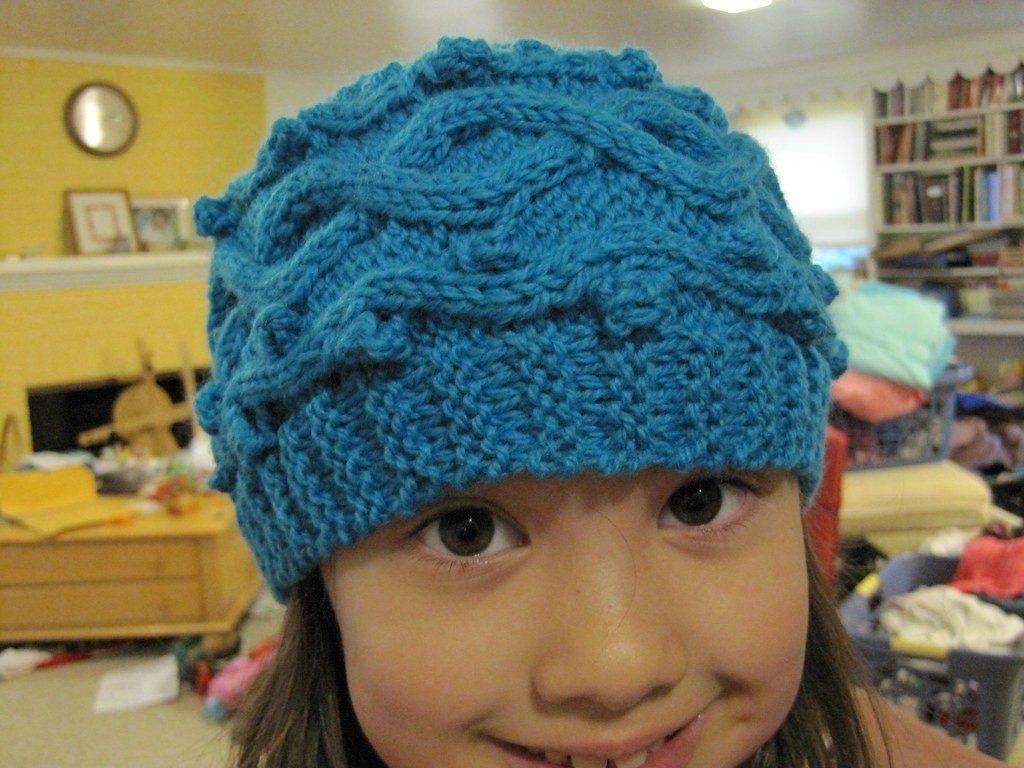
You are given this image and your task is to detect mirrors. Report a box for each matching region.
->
[65,79,139,156]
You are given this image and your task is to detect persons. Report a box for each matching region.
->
[140,209,175,241]
[196,35,1012,768]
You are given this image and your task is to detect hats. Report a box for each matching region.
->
[184,33,851,605]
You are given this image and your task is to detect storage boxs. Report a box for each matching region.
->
[836,359,1024,762]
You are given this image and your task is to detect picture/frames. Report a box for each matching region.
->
[128,195,192,252]
[64,187,138,257]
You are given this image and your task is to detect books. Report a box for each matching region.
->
[880,65,1024,317]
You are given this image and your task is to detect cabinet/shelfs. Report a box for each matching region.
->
[863,61,1024,336]
[1,489,264,648]
